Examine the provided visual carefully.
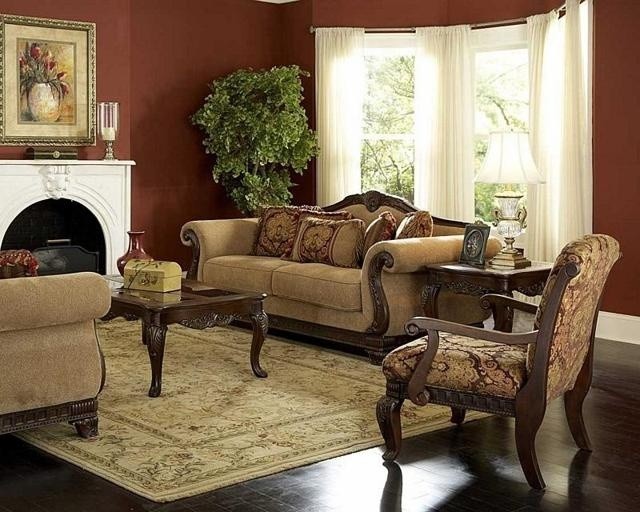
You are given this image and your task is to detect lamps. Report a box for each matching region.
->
[472,132,546,269]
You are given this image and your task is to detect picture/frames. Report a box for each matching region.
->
[0,12,96,146]
[458,219,491,267]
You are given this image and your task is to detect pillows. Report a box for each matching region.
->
[1,249,39,277]
[250,203,434,267]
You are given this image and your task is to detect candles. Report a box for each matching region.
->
[102,127,115,140]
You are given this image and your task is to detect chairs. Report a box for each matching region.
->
[376,233,622,492]
[1,270,112,440]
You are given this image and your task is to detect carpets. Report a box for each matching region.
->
[14,317,498,504]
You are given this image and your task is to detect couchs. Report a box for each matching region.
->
[180,191,505,366]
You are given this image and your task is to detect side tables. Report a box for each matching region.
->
[422,264,551,333]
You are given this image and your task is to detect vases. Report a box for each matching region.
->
[116,229,153,277]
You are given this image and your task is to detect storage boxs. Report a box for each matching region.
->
[123,258,183,292]
